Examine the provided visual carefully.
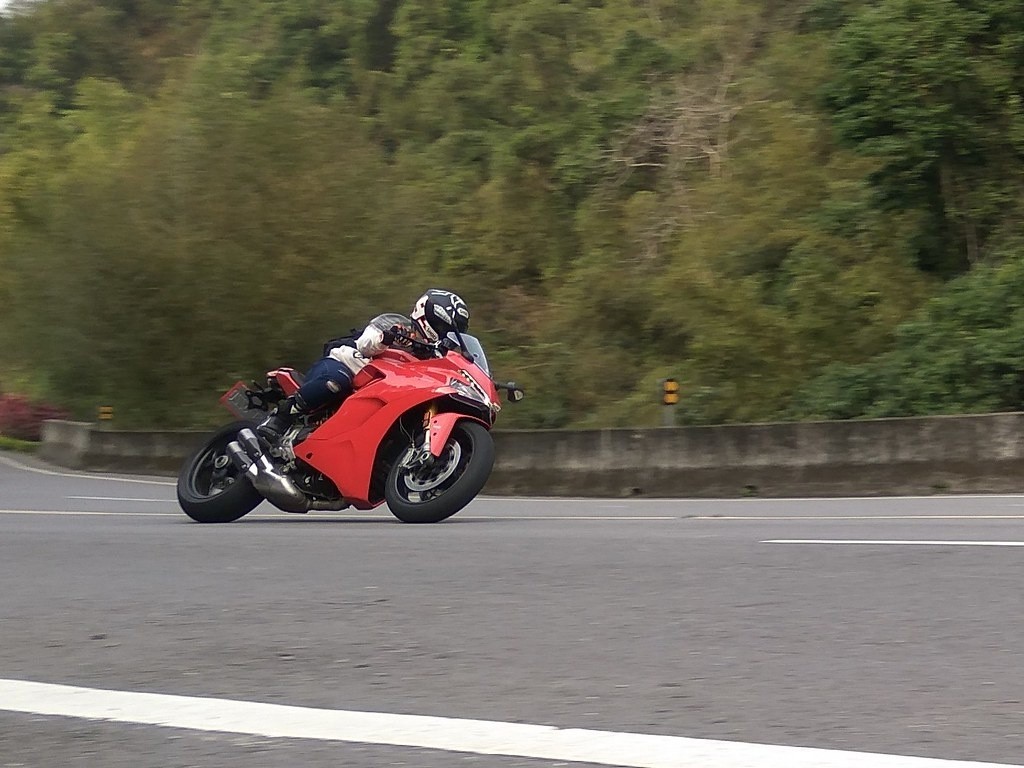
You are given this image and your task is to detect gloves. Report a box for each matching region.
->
[389,324,417,348]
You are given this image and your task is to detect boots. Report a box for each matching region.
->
[256,390,309,443]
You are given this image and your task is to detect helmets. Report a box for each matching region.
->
[410,289,470,356]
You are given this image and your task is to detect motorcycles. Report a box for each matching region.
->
[176,297,524,524]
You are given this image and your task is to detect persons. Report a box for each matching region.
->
[258,287,470,440]
